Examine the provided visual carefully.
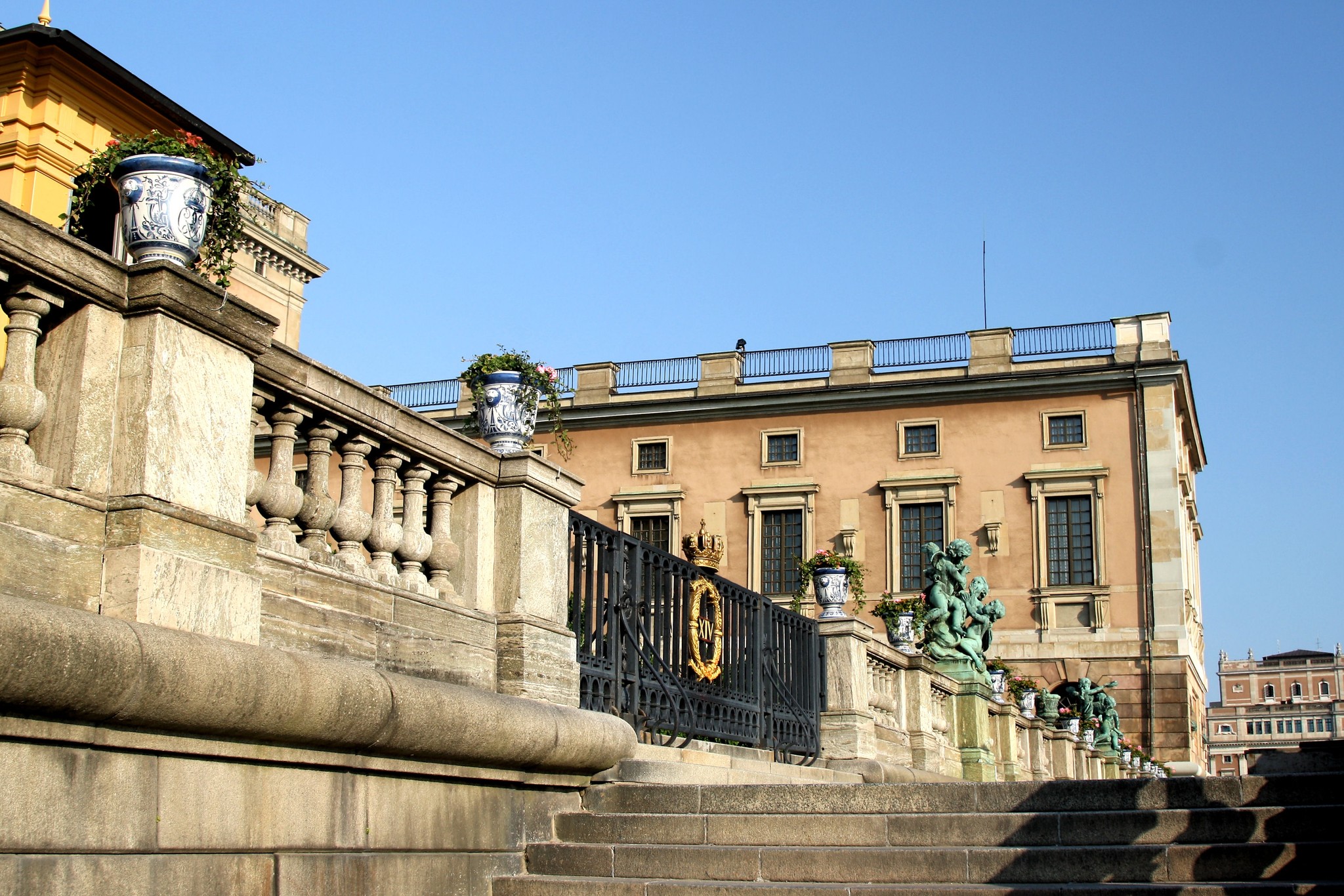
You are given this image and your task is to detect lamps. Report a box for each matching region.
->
[736,339,746,352]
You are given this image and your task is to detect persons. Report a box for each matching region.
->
[1064,676,1124,752]
[920,540,1006,685]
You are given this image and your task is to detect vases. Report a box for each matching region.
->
[468,370,545,454]
[109,152,215,270]
[880,611,916,654]
[1121,749,1131,763]
[811,567,848,618]
[988,670,1006,703]
[1016,688,1035,718]
[1150,763,1158,776]
[1079,729,1094,751]
[1141,760,1151,772]
[1057,717,1080,740]
[1131,756,1140,768]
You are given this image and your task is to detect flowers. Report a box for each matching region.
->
[57,129,283,287]
[1117,736,1176,778]
[456,342,578,464]
[1055,707,1099,741]
[868,590,934,655]
[985,656,1023,682]
[789,550,867,616]
[1006,672,1046,707]
